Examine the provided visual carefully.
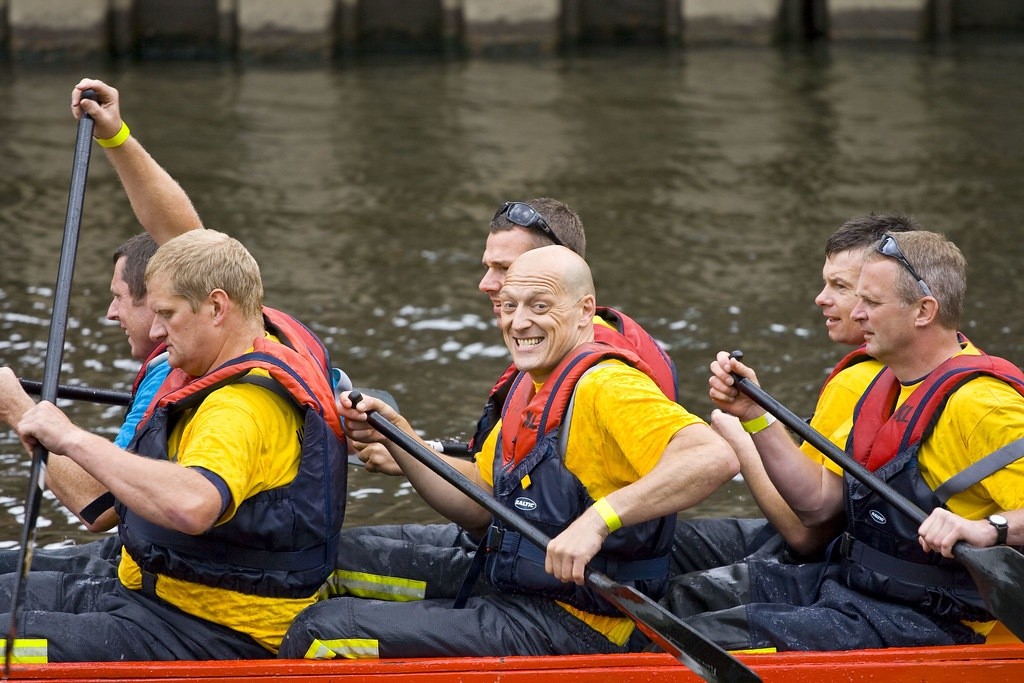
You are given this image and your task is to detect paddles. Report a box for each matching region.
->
[728,348,1024,646]
[16,378,133,407]
[348,389,764,683]
[4,87,101,682]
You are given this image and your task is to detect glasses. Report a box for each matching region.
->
[877,233,934,296]
[492,200,563,246]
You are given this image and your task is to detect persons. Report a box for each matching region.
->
[0,77,1024,662]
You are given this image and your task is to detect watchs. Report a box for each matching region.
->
[984,513,1009,546]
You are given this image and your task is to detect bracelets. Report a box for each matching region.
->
[592,496,623,533]
[92,119,131,149]
[740,412,777,435]
